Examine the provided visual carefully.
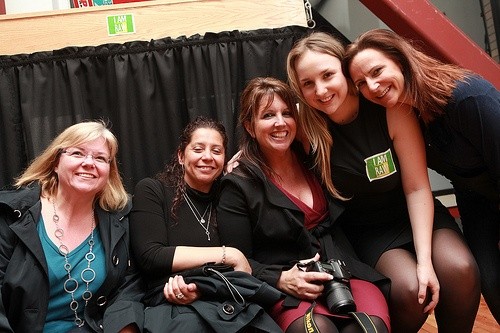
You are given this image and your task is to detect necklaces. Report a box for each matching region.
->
[182,193,213,240]
[181,187,212,224]
[52,193,96,328]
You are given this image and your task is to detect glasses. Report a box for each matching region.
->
[57,149,113,164]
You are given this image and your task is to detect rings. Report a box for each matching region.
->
[176,293,184,299]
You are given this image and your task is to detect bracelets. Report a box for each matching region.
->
[222,245,225,264]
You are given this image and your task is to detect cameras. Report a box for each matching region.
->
[306,259,356,315]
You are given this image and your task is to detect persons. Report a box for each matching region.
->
[216,78,391,333]
[0,122,146,333]
[223,33,482,333]
[343,27,500,326]
[129,117,252,333]
